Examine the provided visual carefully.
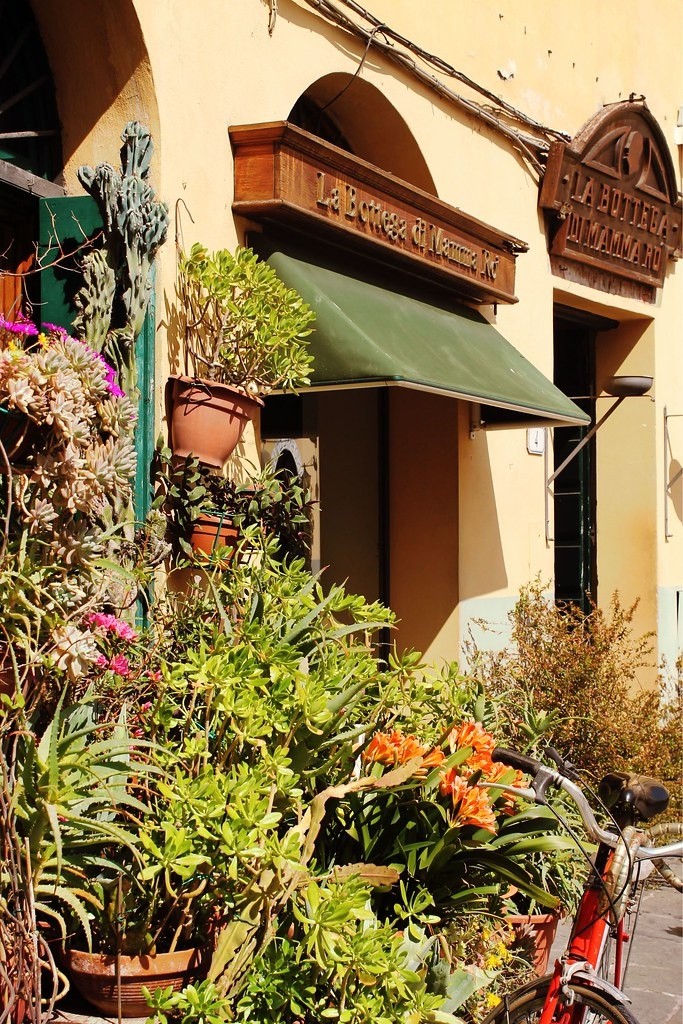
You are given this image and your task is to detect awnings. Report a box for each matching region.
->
[260,227,592,433]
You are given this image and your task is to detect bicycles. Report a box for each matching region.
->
[477,745,683,1024]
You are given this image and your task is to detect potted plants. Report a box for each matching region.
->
[150,435,322,573]
[158,242,319,459]
[73,632,336,1017]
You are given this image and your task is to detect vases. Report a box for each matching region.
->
[495,884,518,916]
[439,907,570,979]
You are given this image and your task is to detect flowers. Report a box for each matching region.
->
[361,720,531,835]
